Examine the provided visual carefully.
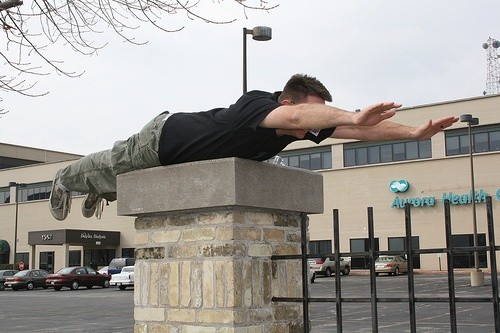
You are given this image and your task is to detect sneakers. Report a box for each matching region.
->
[80,192,104,220]
[49,168,73,222]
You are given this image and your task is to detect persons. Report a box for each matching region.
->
[48,74,459,221]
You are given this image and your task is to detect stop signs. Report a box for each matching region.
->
[19,262,24,270]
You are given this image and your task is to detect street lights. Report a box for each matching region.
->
[8,182,19,268]
[242,26,272,95]
[461,113,480,272]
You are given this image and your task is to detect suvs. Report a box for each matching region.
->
[97,257,135,274]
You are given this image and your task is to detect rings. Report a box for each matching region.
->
[441,125,444,128]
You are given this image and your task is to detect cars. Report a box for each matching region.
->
[0,270,20,291]
[374,254,409,276]
[46,266,110,290]
[3,269,48,291]
[109,265,134,291]
[308,257,350,277]
